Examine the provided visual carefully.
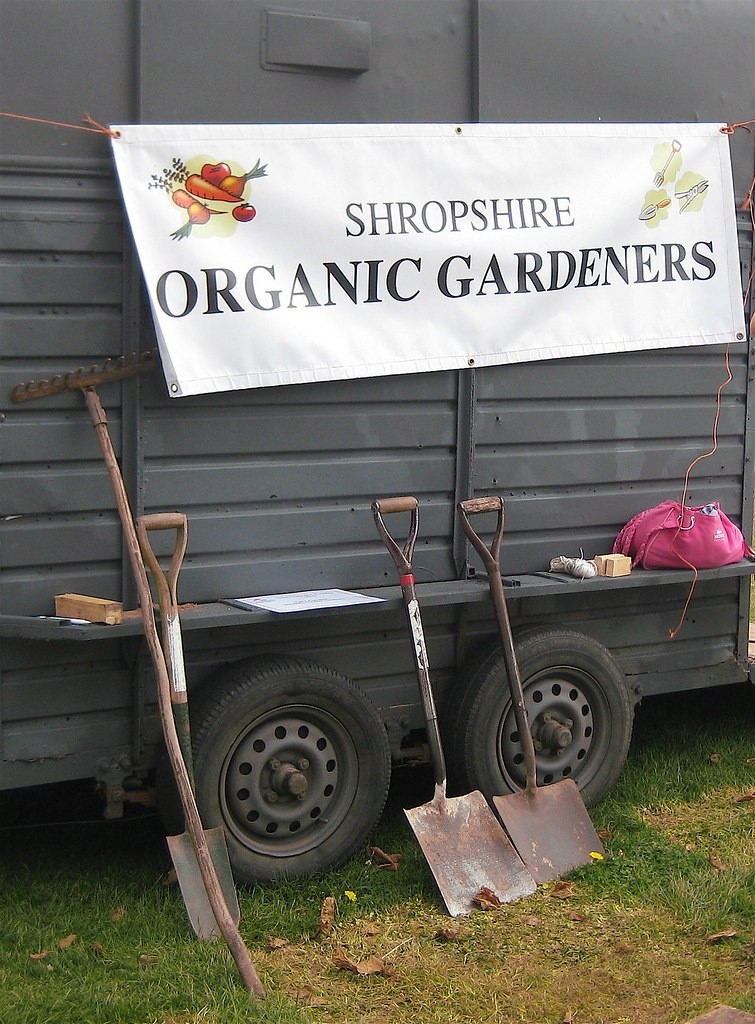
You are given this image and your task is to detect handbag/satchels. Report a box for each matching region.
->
[611,500,746,571]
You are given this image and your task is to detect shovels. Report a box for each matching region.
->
[372,496,537,917]
[137,511,241,940]
[457,496,606,885]
